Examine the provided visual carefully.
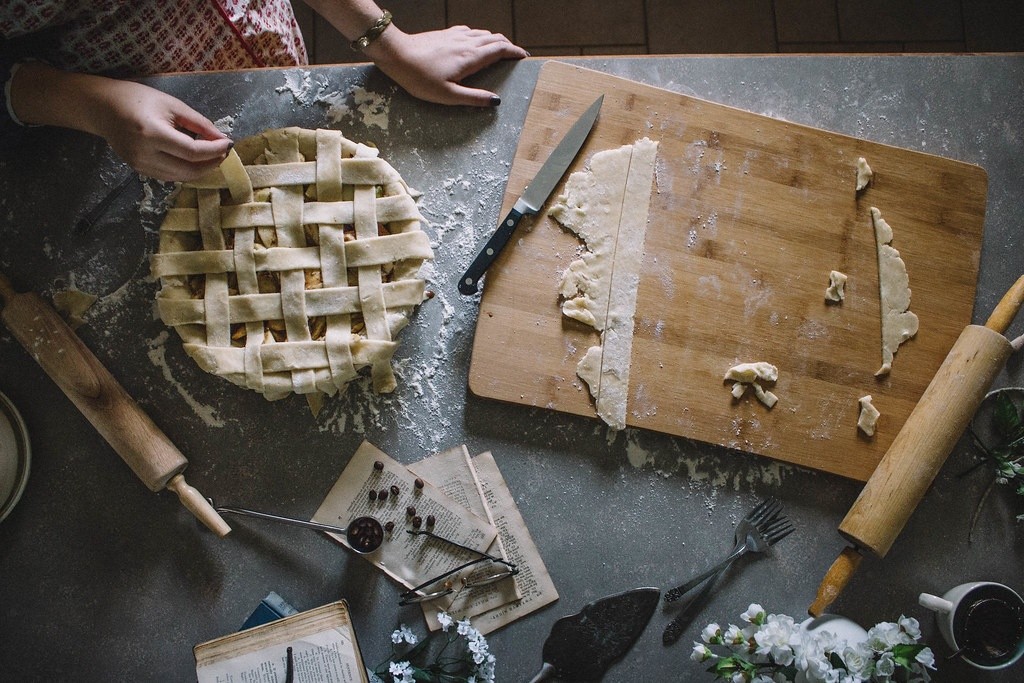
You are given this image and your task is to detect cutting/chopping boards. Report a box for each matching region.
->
[468,60,990,480]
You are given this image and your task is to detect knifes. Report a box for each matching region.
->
[456,94,606,294]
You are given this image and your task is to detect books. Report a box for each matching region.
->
[193,593,370,683]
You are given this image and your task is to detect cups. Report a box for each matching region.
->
[919,581,1024,670]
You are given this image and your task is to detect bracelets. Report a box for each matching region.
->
[351,9,393,53]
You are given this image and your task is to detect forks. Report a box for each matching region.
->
[662,496,784,644]
[664,515,796,603]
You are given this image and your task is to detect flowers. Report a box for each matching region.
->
[370,611,497,683]
[956,392,1024,544]
[690,602,939,683]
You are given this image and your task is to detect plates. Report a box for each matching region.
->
[0,392,31,522]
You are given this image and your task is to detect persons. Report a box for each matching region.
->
[0,0,531,182]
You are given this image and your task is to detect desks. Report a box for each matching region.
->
[0,50,1024,683]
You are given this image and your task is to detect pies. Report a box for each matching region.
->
[147,129,435,400]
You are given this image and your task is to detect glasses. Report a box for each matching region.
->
[397,530,518,606]
